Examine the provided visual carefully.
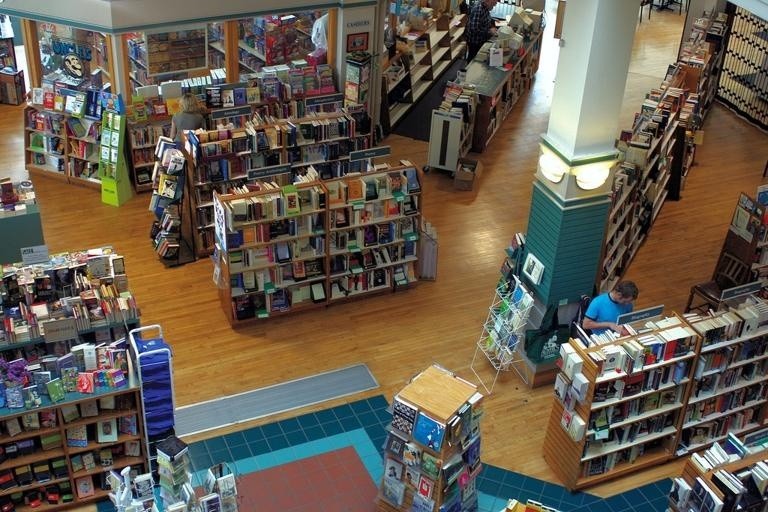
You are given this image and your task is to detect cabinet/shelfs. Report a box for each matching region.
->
[379,3,468,139]
[469,253,540,398]
[209,160,423,332]
[426,16,544,173]
[24,241,145,331]
[685,181,768,313]
[547,296,767,492]
[1,175,241,511]
[147,104,373,268]
[372,368,485,511]
[596,11,729,294]
[25,12,346,202]
[667,426,767,512]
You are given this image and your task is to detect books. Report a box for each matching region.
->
[376,367,488,512]
[23,1,547,190]
[1,245,240,511]
[598,11,735,295]
[554,174,766,510]
[145,155,437,324]
[503,496,556,511]
[486,231,544,371]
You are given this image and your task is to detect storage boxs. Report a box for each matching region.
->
[451,159,483,189]
